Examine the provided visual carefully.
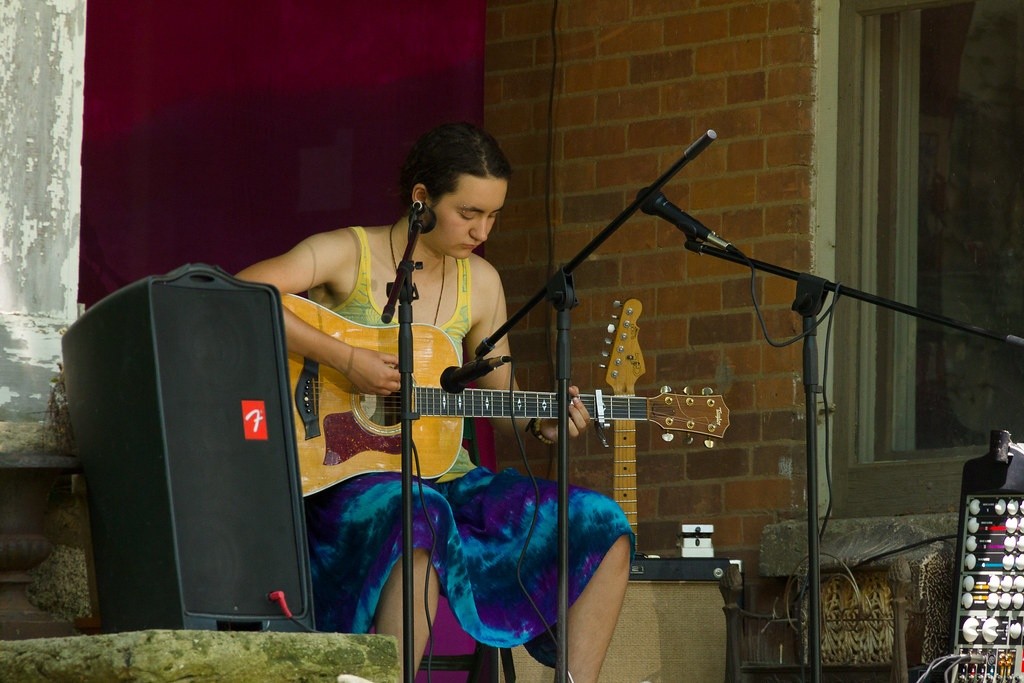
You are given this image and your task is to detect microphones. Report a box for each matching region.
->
[440,355,512,393]
[636,187,752,265]
[407,202,437,235]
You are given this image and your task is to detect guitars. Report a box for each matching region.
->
[595,292,648,563]
[273,289,733,499]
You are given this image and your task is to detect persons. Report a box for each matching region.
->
[229,117,642,681]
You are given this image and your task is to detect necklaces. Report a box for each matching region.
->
[390,223,447,330]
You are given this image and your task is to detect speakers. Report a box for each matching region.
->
[60,264,317,632]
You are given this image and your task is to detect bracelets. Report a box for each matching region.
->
[526,411,556,446]
